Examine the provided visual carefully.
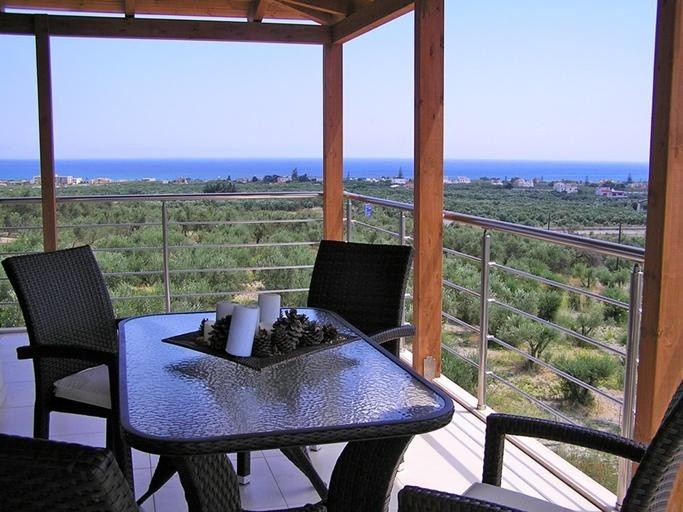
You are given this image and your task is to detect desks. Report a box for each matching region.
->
[113,307,457,511]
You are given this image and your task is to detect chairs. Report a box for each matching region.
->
[396,377,682,511]
[1,243,137,498]
[228,238,417,486]
[1,417,140,512]
